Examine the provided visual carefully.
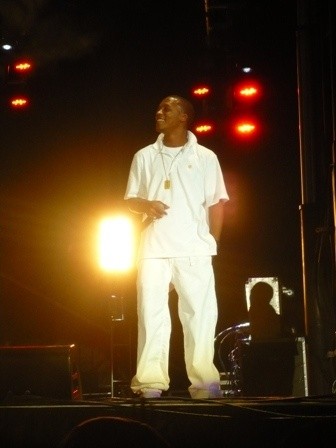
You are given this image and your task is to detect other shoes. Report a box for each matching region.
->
[195,390,221,399]
[141,388,160,398]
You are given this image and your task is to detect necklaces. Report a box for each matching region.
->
[159,148,187,189]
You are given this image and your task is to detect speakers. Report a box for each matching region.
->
[0,343,84,406]
[237,336,296,398]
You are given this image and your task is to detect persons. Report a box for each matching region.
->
[121,94,231,399]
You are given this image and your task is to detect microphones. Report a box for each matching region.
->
[140,216,154,231]
